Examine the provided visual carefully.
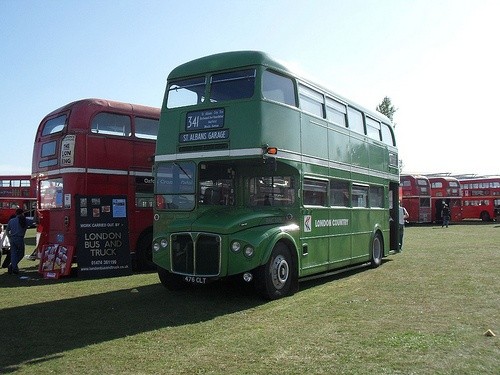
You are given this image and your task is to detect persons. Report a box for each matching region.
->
[399,200,409,250]
[435,200,451,228]
[5,208,37,274]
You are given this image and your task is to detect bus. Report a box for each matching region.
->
[398,172,500,225]
[0,175,37,224]
[31,97,163,274]
[152,49,405,302]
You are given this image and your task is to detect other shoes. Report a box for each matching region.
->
[13,271,20,275]
[8,265,12,274]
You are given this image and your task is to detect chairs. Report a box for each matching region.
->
[203,188,224,203]
